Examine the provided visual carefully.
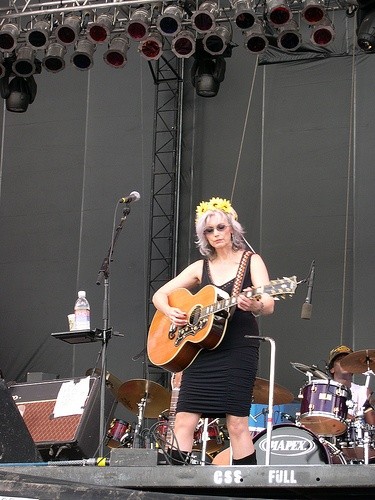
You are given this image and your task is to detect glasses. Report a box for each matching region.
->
[206,223,230,233]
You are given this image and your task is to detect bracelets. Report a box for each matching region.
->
[251,301,265,318]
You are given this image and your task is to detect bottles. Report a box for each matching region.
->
[74,290,90,331]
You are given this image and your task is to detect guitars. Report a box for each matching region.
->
[145,274,300,375]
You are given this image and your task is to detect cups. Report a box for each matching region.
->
[68,314,75,331]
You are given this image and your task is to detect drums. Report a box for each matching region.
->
[190,448,213,465]
[157,415,225,454]
[105,419,132,448]
[332,423,375,462]
[299,379,353,438]
[252,423,347,465]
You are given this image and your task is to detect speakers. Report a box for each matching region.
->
[0,376,118,463]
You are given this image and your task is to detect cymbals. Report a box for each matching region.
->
[341,349,375,374]
[251,378,294,405]
[117,378,172,419]
[86,368,122,401]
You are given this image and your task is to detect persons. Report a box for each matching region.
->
[147,197,275,465]
[327,345,372,415]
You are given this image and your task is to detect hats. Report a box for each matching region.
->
[327,345,354,367]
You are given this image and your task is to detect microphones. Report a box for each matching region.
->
[120,191,141,203]
[301,262,316,320]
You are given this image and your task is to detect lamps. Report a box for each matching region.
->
[0,0,375,113]
